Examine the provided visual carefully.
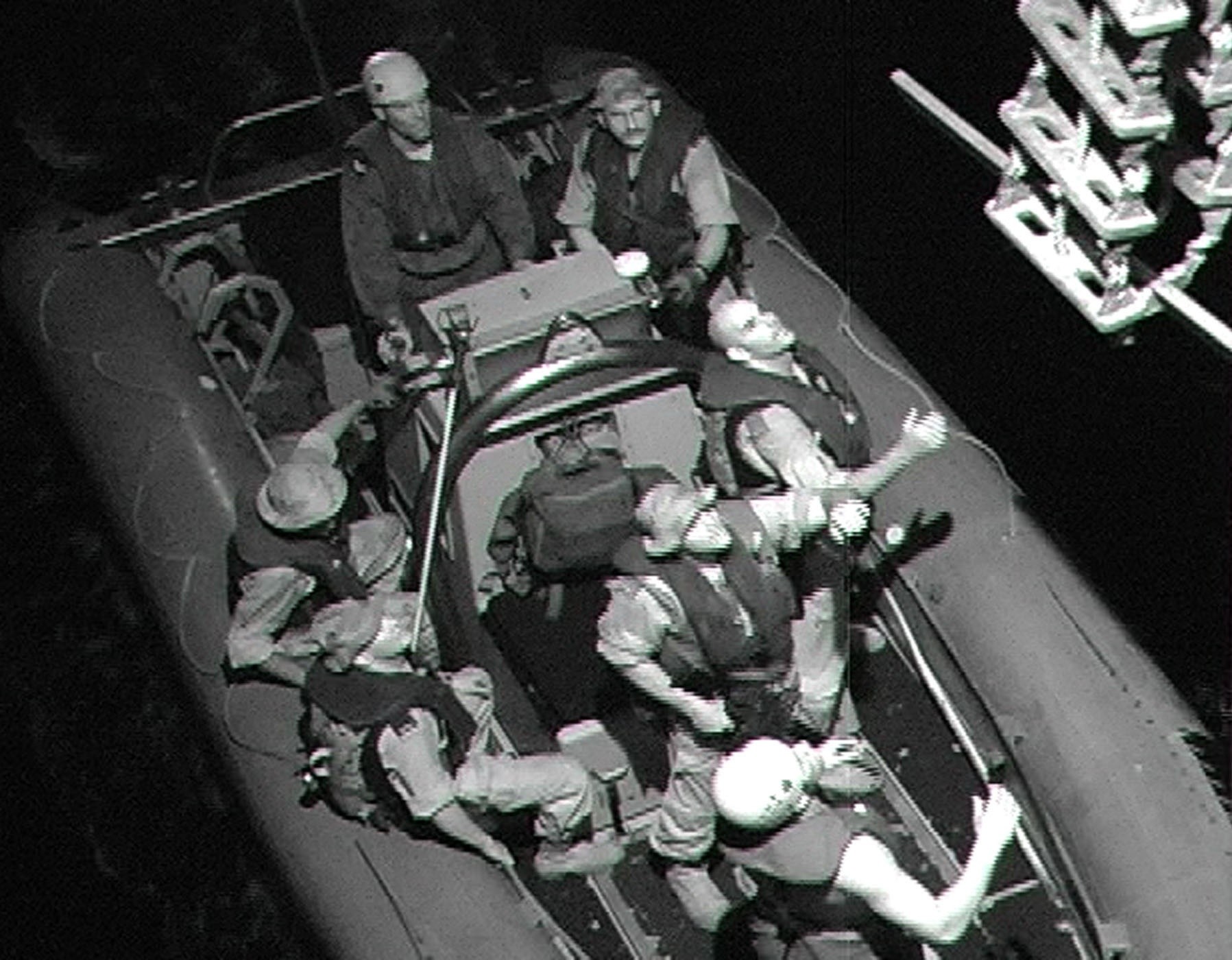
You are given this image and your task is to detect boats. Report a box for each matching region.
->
[1,44,1232,960]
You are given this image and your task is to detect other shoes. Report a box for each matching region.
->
[537,841,624,876]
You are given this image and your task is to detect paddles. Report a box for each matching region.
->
[857,550,1060,904]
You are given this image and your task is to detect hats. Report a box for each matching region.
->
[319,600,382,671]
[255,463,348,531]
[635,484,716,555]
[589,67,662,108]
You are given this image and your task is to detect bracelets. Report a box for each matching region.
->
[686,261,711,280]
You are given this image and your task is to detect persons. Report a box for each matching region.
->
[706,737,1021,960]
[298,599,623,878]
[555,69,737,341]
[225,375,443,686]
[696,298,948,734]
[340,49,537,363]
[596,483,873,931]
